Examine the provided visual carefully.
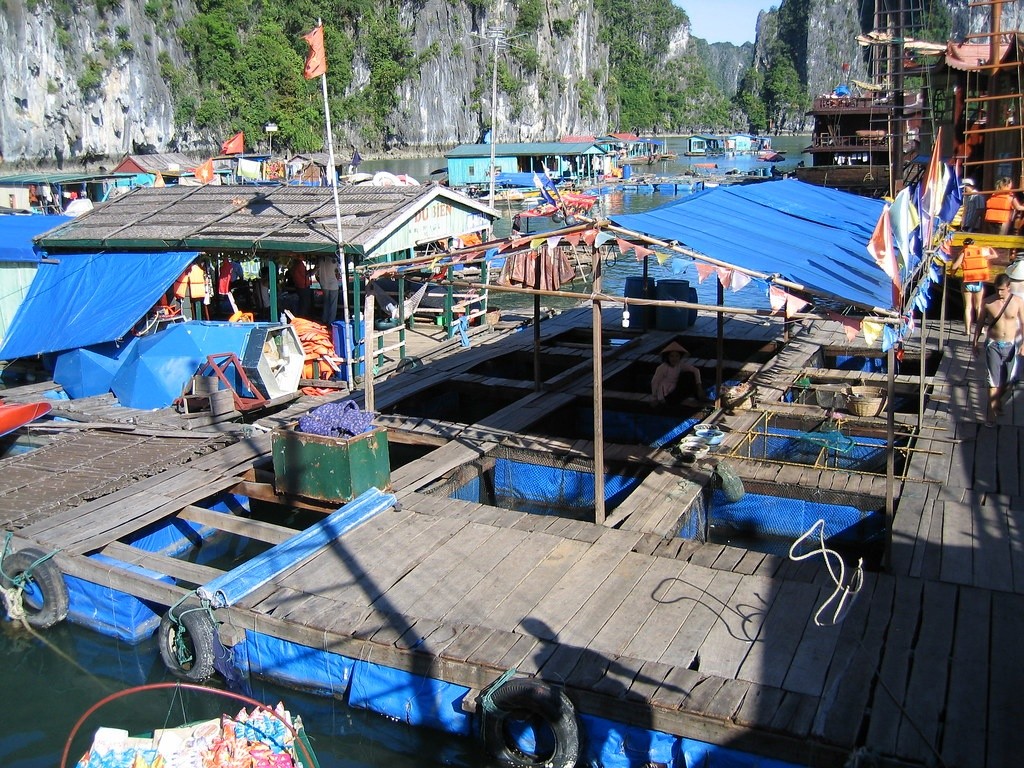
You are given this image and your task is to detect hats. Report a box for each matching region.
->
[959,179,974,188]
[659,342,689,360]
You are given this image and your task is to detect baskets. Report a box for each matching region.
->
[486,306,501,325]
[841,386,888,417]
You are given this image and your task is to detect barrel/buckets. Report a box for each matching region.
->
[331,319,365,381]
[623,165,632,179]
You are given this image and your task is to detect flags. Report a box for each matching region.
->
[352,150,364,168]
[866,203,902,296]
[222,131,243,155]
[154,172,166,188]
[194,157,215,184]
[300,24,327,79]
[889,123,963,283]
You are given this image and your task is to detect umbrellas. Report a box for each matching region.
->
[756,151,786,168]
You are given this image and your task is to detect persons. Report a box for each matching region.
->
[650,342,705,424]
[284,253,319,319]
[797,161,805,168]
[831,90,840,104]
[951,177,1024,335]
[770,165,788,177]
[315,255,340,329]
[254,266,272,323]
[229,255,244,290]
[972,273,1024,427]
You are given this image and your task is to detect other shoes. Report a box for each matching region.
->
[985,420,994,428]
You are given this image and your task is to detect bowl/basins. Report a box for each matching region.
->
[679,423,725,460]
